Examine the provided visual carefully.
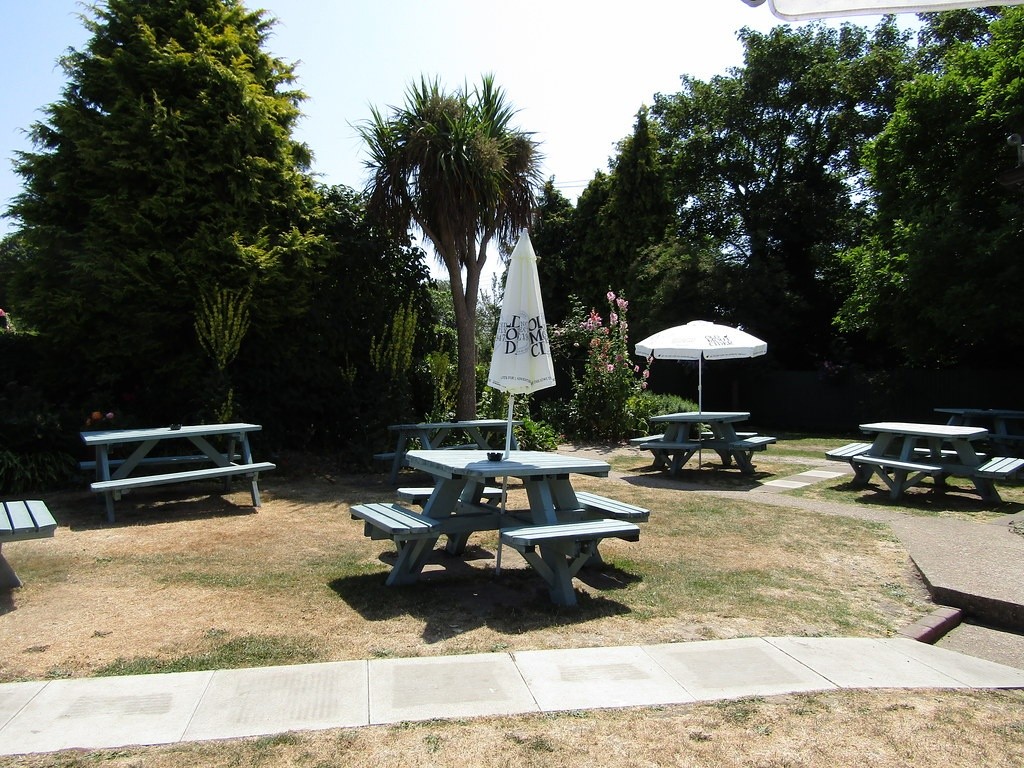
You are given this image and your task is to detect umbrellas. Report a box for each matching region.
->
[487,228,556,575]
[635,320,767,470]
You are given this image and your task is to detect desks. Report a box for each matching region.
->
[933,407,1023,466]
[382,451,610,607]
[653,412,755,477]
[849,421,1001,510]
[81,421,261,524]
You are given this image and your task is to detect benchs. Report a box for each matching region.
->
[0,432,1024,541]
